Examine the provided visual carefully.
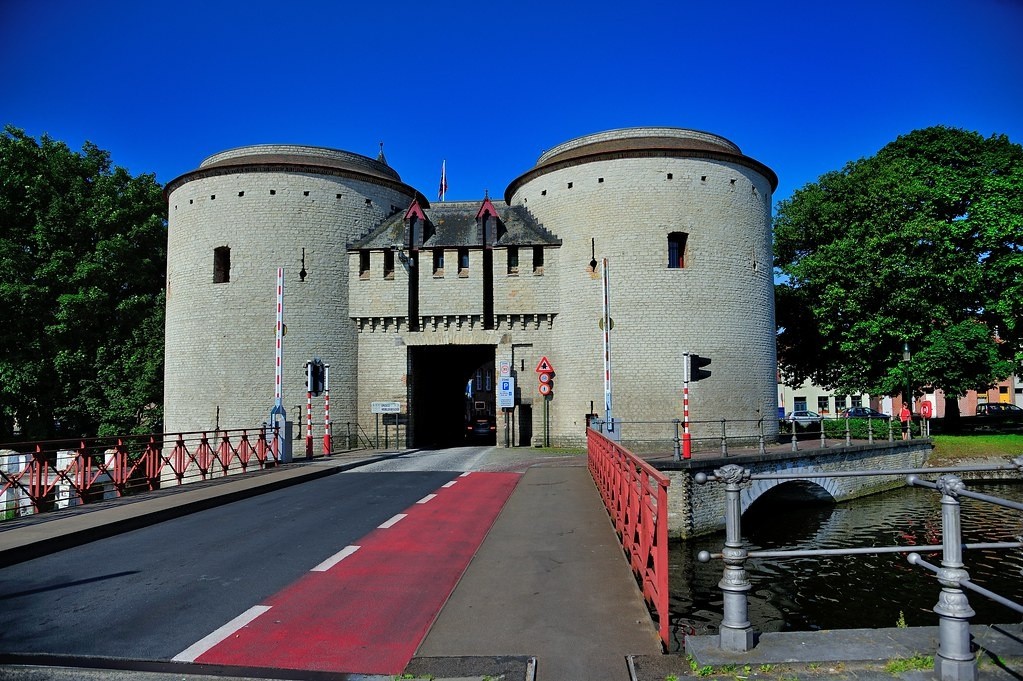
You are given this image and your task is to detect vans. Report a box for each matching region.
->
[975,402,1023,425]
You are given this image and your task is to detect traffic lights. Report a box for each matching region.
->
[690,354,712,381]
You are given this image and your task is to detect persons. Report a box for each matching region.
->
[899,402,911,440]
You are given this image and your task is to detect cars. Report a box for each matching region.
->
[784,411,834,429]
[466,415,496,446]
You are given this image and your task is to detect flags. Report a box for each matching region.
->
[438,167,447,201]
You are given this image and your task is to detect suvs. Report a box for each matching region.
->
[839,406,890,422]
